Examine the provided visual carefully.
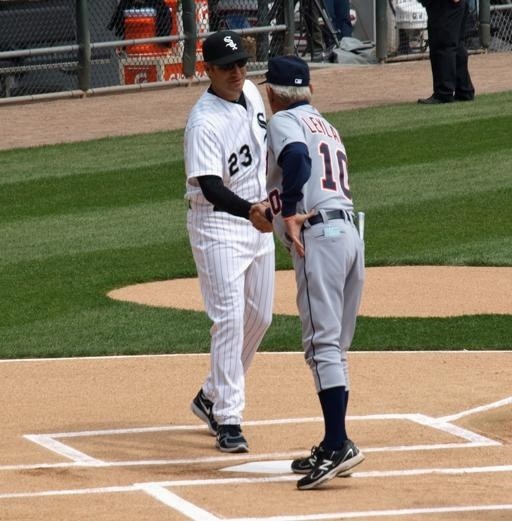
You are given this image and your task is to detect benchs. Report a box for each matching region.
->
[1,6,112,97]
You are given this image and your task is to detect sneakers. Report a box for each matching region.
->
[290,438,365,490]
[190,387,251,454]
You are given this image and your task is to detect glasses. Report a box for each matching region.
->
[217,57,249,71]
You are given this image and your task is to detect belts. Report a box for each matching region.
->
[301,210,355,231]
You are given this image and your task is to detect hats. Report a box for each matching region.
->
[202,31,253,66]
[257,54,311,88]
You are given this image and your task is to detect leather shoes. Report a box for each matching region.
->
[418,95,443,105]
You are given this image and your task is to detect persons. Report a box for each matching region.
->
[415,1,477,104]
[257,1,353,63]
[247,55,369,493]
[181,30,276,453]
